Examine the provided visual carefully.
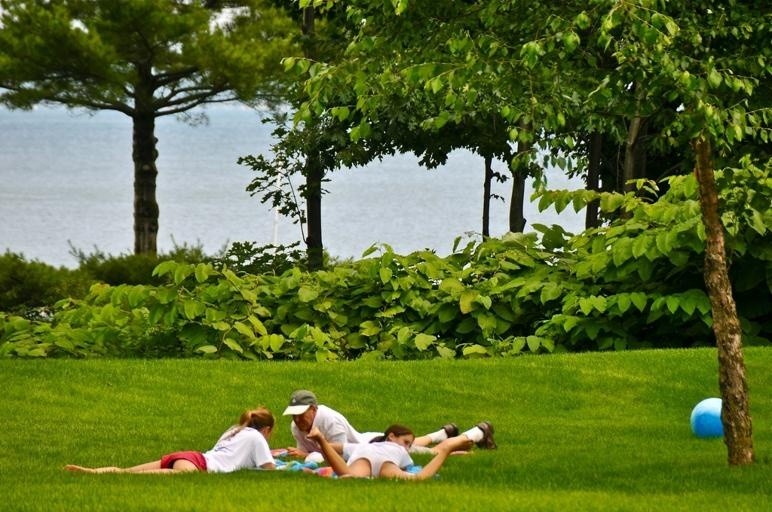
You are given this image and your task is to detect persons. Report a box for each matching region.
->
[64,407,276,474]
[306,425,474,481]
[282,390,497,459]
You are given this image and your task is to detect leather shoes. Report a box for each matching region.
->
[475,421,497,449]
[440,422,458,438]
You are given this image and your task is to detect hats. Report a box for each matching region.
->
[282,389,317,415]
